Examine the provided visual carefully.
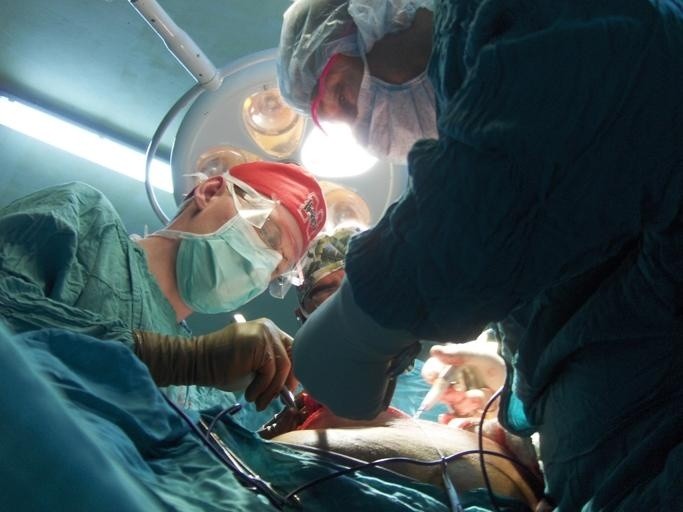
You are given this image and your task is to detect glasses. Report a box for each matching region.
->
[229,183,294,300]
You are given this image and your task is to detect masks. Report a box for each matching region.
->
[173,214,282,317]
[348,29,440,165]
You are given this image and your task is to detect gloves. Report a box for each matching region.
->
[289,274,424,425]
[132,318,301,412]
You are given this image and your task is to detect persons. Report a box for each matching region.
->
[260,398,551,512]
[292,233,396,383]
[274,0,682,509]
[1,163,327,451]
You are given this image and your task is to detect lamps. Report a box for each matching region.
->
[127,0,394,234]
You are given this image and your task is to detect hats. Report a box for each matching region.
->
[188,159,328,267]
[295,223,373,303]
